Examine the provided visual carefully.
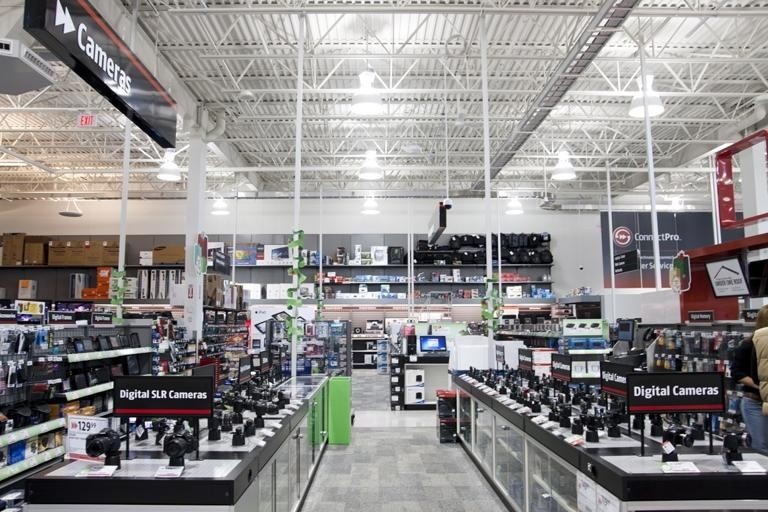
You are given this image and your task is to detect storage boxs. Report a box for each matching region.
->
[152,242,185,264]
[18,279,38,299]
[0,231,128,266]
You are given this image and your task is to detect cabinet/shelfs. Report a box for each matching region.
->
[204,233,555,301]
[203,303,251,385]
[455,384,768,510]
[0,326,152,486]
[25,380,331,511]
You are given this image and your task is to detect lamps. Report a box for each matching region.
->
[155,149,182,183]
[628,75,665,117]
[350,71,385,120]
[361,191,381,215]
[356,150,385,181]
[551,151,577,180]
[504,191,525,216]
[210,193,230,216]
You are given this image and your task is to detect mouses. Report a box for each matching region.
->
[631,346,637,352]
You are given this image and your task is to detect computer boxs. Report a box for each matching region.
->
[406,334,416,354]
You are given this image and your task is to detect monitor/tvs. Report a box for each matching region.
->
[746,259,768,298]
[704,257,751,299]
[238,354,252,384]
[617,317,635,354]
[270,246,290,261]
[258,349,271,374]
[191,363,217,381]
[420,335,447,356]
[252,311,305,349]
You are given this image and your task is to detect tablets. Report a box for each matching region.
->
[73,332,141,389]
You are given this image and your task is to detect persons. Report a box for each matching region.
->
[728,304,767,455]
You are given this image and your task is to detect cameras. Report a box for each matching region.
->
[723,429,752,464]
[134,380,292,466]
[86,429,123,468]
[469,365,710,463]
